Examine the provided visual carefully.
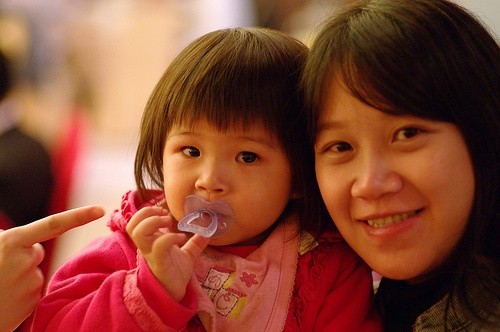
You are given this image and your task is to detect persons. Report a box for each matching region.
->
[30,26,383,332]
[295,0,500,332]
[0,17,105,332]
